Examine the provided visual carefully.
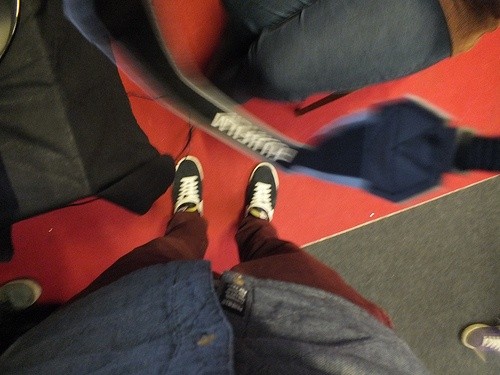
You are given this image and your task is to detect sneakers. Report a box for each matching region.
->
[0,279,41,312]
[244,162,279,224]
[460,323,500,354]
[174,156,204,216]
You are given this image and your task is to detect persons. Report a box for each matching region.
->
[0,157,432,375]
[209,0,500,99]
